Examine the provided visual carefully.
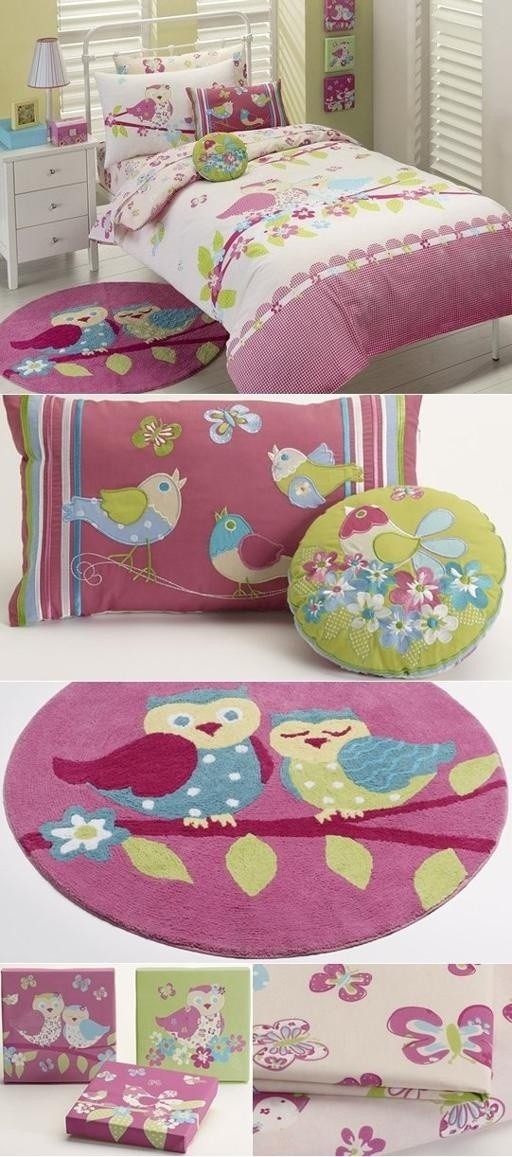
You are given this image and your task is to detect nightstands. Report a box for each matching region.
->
[0,139,101,290]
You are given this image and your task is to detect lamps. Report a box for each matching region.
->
[26,37,70,130]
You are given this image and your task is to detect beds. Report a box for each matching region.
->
[80,8,512,394]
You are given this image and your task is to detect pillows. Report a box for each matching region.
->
[92,60,236,168]
[192,132,249,180]
[289,486,507,681]
[184,81,290,138]
[112,38,249,97]
[0,395,423,624]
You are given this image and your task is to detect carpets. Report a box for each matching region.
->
[0,280,227,394]
[5,684,508,962]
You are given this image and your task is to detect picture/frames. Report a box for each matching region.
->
[12,98,39,128]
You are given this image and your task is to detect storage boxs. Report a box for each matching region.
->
[0,119,49,150]
[52,118,87,145]
